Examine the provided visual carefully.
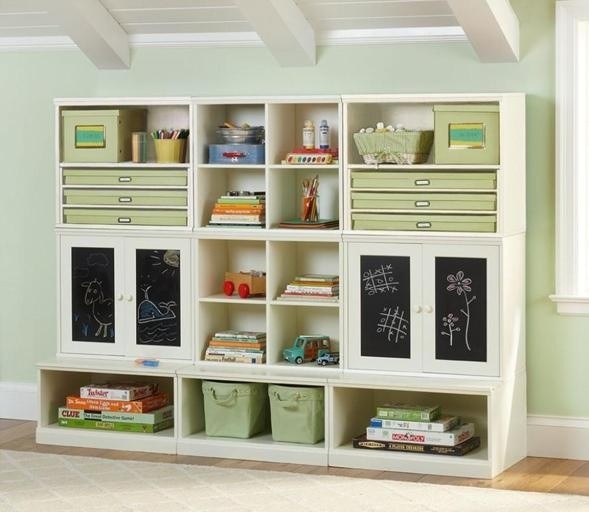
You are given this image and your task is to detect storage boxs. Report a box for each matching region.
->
[61,109,147,163]
[430,105,500,166]
[200,380,265,438]
[269,384,325,446]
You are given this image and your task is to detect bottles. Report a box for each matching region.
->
[303,120,329,149]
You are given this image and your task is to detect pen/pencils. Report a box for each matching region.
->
[150,128,189,139]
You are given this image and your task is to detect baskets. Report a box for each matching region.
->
[353,130,434,164]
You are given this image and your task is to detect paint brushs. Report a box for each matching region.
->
[301,174,320,222]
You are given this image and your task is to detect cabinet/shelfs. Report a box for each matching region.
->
[333,376,526,475]
[37,366,177,455]
[193,103,342,231]
[348,235,526,376]
[53,103,194,231]
[55,237,192,362]
[200,239,341,370]
[178,369,329,466]
[346,99,526,235]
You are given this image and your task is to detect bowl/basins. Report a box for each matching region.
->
[218,127,263,144]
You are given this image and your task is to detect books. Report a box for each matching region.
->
[277,275,339,302]
[205,191,265,229]
[54,379,172,434]
[205,330,266,364]
[353,400,481,456]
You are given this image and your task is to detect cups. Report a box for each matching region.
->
[301,195,320,222]
[131,131,149,163]
